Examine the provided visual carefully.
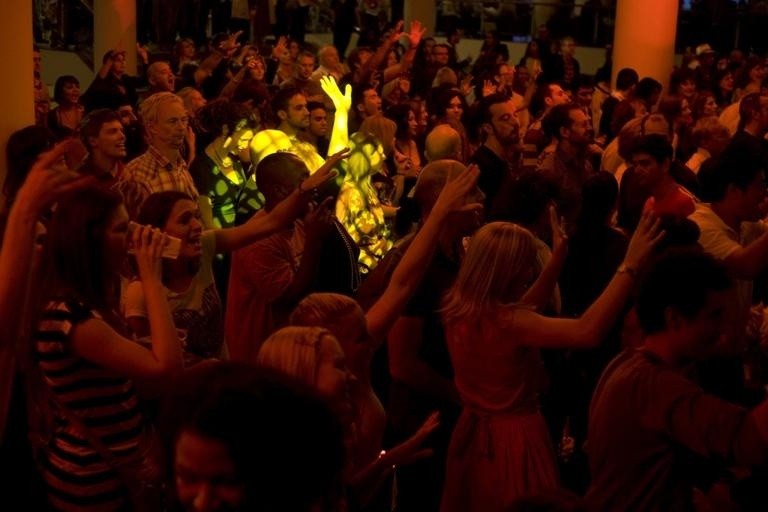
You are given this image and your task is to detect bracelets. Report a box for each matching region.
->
[299,181,314,195]
[378,450,396,471]
[408,44,417,49]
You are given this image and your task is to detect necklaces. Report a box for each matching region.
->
[395,141,412,160]
[64,108,75,123]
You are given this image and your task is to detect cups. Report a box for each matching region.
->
[125,221,181,261]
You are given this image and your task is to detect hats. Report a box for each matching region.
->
[695,43,715,55]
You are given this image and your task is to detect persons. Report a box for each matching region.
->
[0,19,768,512]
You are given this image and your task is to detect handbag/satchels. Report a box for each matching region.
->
[108,421,168,512]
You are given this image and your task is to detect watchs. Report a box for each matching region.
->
[618,263,639,280]
